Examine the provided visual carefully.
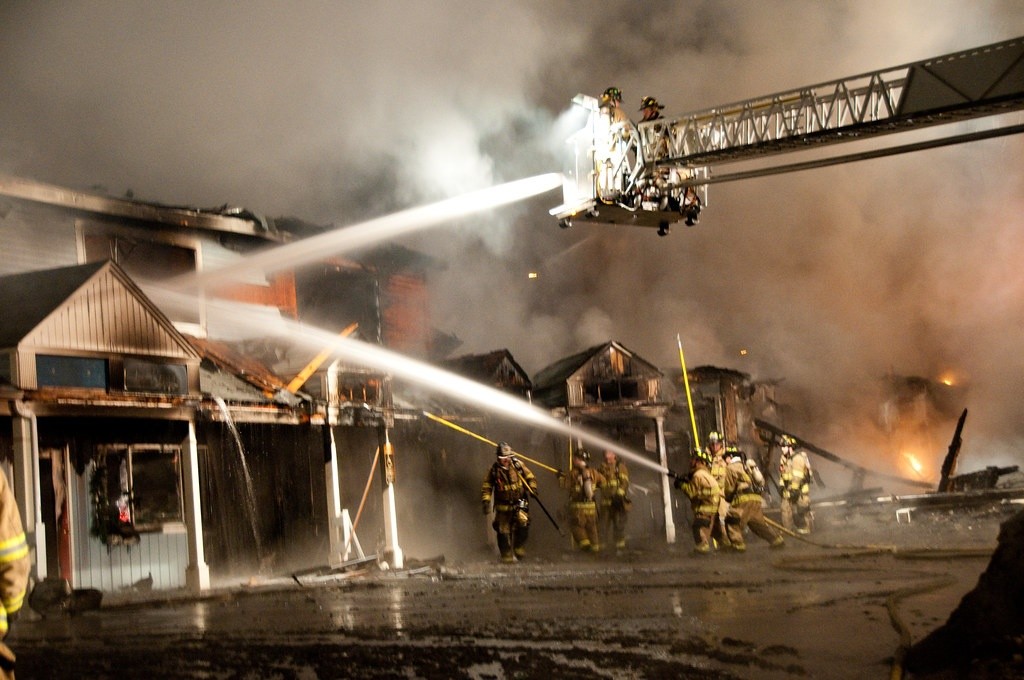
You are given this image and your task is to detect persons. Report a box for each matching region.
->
[778,437,813,534]
[557,448,629,552]
[598,86,671,205]
[673,431,786,552]
[0,466,32,680]
[480,441,536,563]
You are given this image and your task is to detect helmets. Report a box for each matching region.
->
[574,448,591,463]
[779,435,797,446]
[637,95,665,112]
[496,442,515,458]
[604,87,625,103]
[706,430,724,444]
[688,446,712,464]
[721,445,739,460]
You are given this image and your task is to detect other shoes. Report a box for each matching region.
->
[779,526,797,535]
[578,545,592,555]
[798,527,814,535]
[769,536,785,549]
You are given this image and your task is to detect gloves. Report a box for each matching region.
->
[530,487,539,499]
[792,490,799,505]
[778,486,784,497]
[556,469,562,478]
[612,495,624,513]
[674,478,682,490]
[482,501,491,515]
